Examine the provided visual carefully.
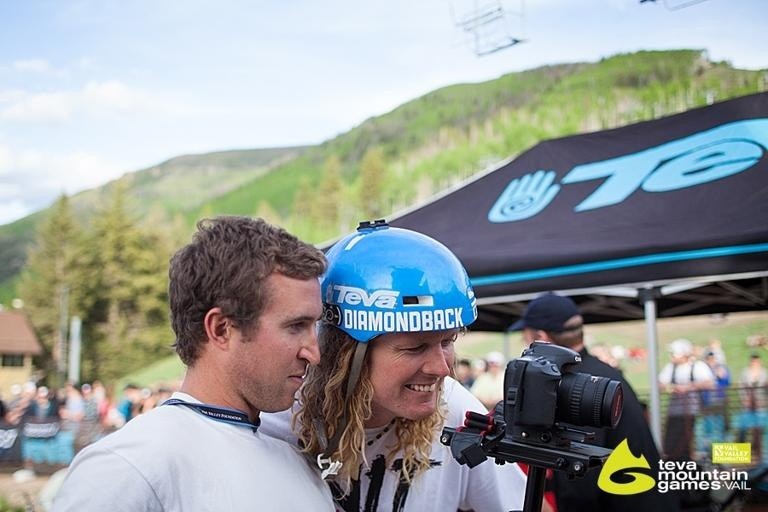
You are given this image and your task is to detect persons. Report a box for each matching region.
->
[733,348,768,454]
[501,291,667,511]
[53,215,338,512]
[255,216,530,512]
[658,338,715,461]
[0,374,174,484]
[451,349,507,409]
[698,346,729,436]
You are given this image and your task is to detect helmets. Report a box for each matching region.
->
[320,221,478,343]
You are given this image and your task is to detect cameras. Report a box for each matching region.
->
[503,339,624,450]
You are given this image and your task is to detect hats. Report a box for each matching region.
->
[508,294,583,333]
